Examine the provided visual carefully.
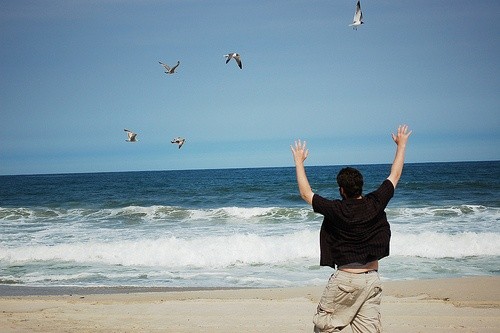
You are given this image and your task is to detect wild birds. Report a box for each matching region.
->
[348,0,364,31]
[124,129,139,142]
[170,136,185,149]
[158,61,180,74]
[225,53,243,69]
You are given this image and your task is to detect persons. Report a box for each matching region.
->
[290,124,412,333]
